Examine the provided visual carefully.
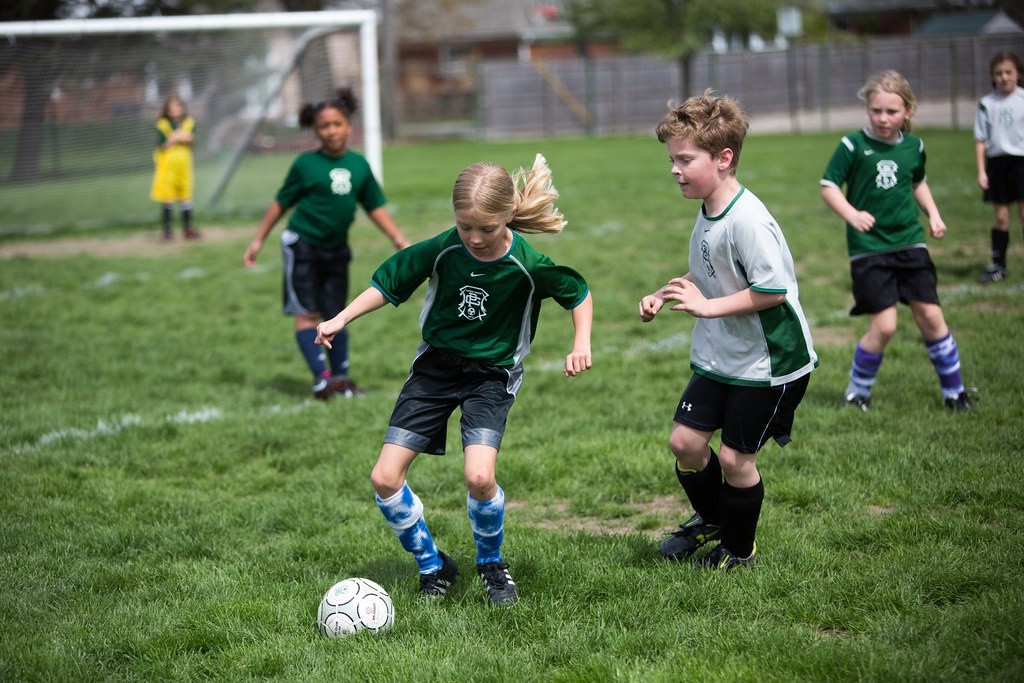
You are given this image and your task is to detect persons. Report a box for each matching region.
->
[973,52,1024,283]
[150,96,200,242]
[314,151,593,604]
[245,87,412,400]
[637,87,819,564]
[820,69,971,409]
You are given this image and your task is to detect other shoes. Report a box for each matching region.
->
[317,379,361,400]
[849,394,870,409]
[983,265,1007,284]
[944,392,974,411]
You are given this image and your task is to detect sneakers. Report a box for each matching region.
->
[698,542,757,572]
[660,514,723,560]
[475,561,518,609]
[418,552,459,607]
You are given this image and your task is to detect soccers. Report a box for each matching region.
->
[317,577,396,638]
